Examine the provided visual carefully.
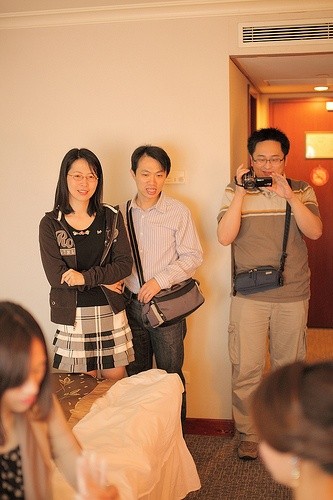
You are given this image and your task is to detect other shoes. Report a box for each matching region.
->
[237,441,259,460]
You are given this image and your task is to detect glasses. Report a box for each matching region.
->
[67,173,99,182]
[252,155,285,166]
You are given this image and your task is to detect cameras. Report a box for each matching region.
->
[241,167,272,190]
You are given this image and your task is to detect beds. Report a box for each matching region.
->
[46,372,201,500]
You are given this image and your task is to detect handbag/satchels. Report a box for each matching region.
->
[146,276,205,330]
[233,264,284,295]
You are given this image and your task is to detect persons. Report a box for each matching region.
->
[39,148,135,381]
[113,146,203,438]
[0,300,119,500]
[250,361,333,500]
[217,128,322,459]
